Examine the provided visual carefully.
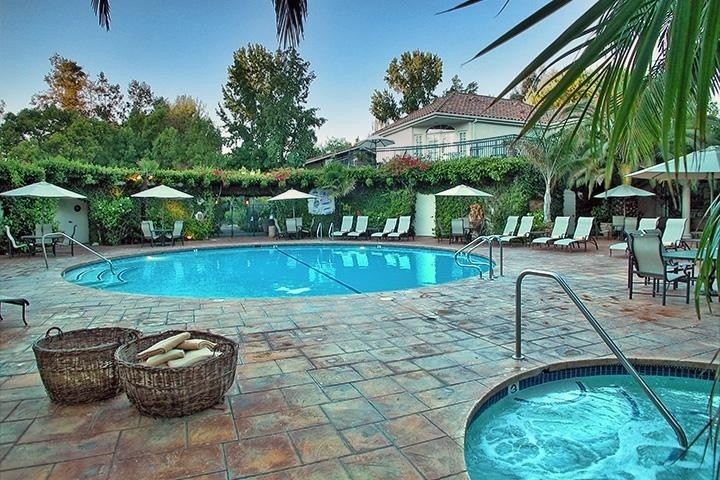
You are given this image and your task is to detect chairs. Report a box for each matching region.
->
[284,213,415,248]
[285,248,410,271]
[610,214,720,304]
[436,215,599,251]
[4,219,77,260]
[140,219,186,247]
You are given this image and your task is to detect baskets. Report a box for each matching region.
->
[31,326,142,404]
[113,330,238,417]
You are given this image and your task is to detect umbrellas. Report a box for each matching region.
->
[357,134,395,163]
[591,182,657,218]
[266,187,317,219]
[433,183,494,218]
[623,142,719,216]
[129,184,196,229]
[0,178,88,235]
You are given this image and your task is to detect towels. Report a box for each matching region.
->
[137,331,222,370]
